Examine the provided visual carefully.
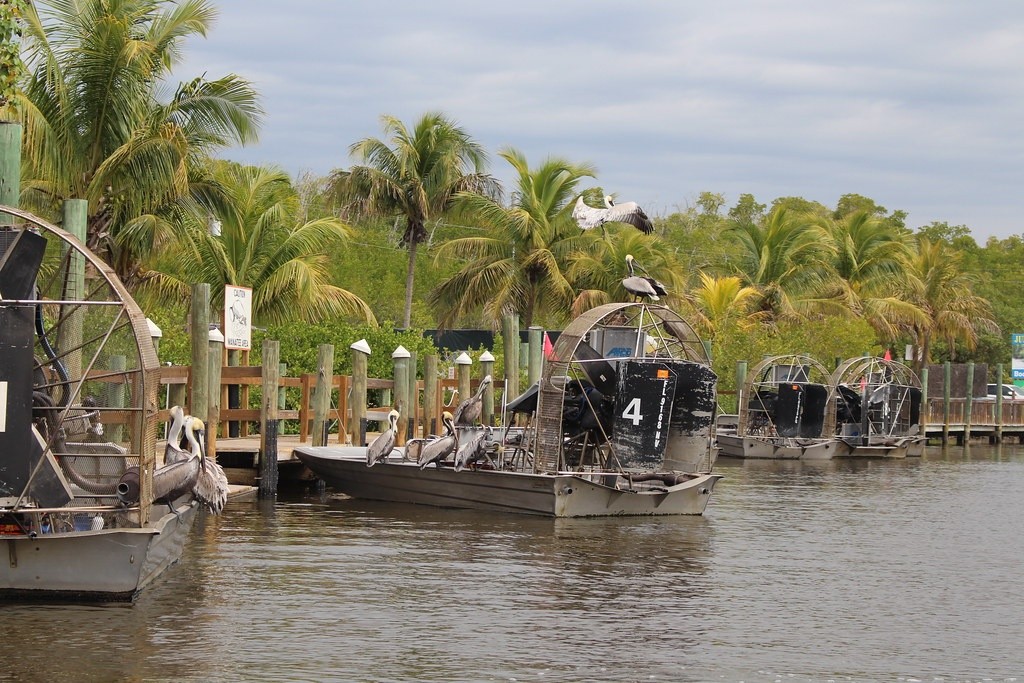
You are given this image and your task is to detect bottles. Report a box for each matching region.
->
[91,511,105,531]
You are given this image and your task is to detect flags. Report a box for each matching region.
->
[885,349,891,360]
[544,333,557,367]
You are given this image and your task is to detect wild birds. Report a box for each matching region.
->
[453,375,491,432]
[366,409,400,467]
[152,417,207,525]
[418,411,459,471]
[455,426,494,473]
[622,254,669,303]
[163,405,229,516]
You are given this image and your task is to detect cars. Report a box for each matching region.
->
[988,384,1024,400]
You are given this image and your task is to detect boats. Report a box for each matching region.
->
[1,207,198,602]
[293,301,725,515]
[716,353,840,459]
[829,356,928,459]
